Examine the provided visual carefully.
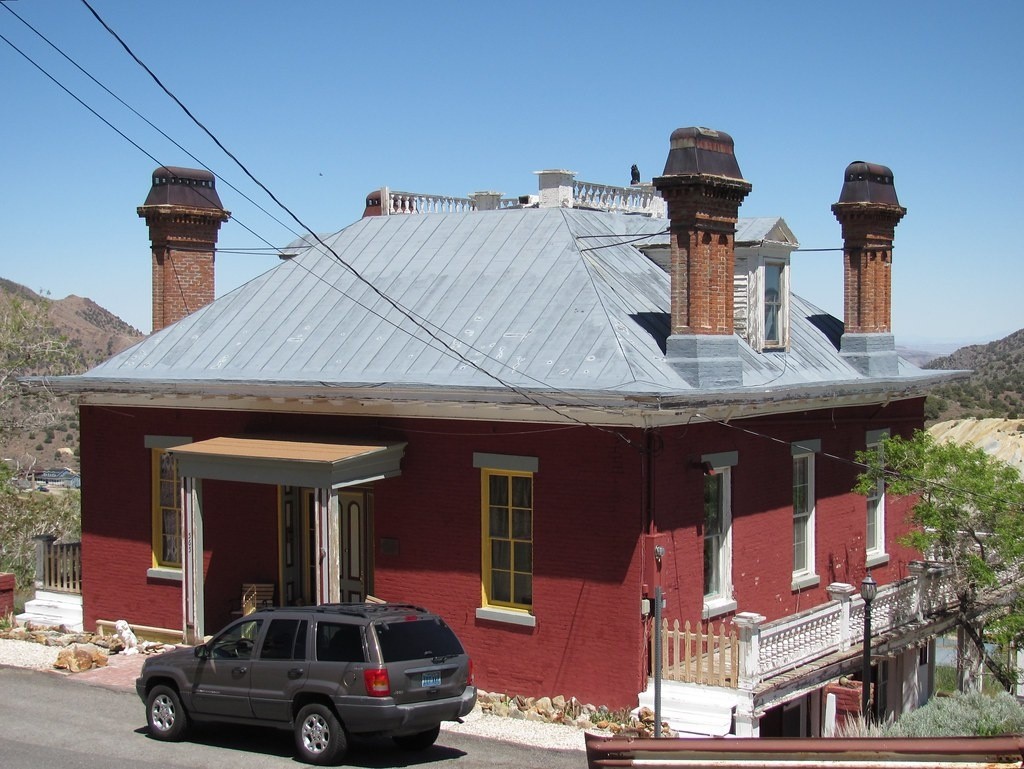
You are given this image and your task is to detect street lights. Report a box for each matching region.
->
[860,567,877,730]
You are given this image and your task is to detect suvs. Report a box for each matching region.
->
[136,601,480,767]
